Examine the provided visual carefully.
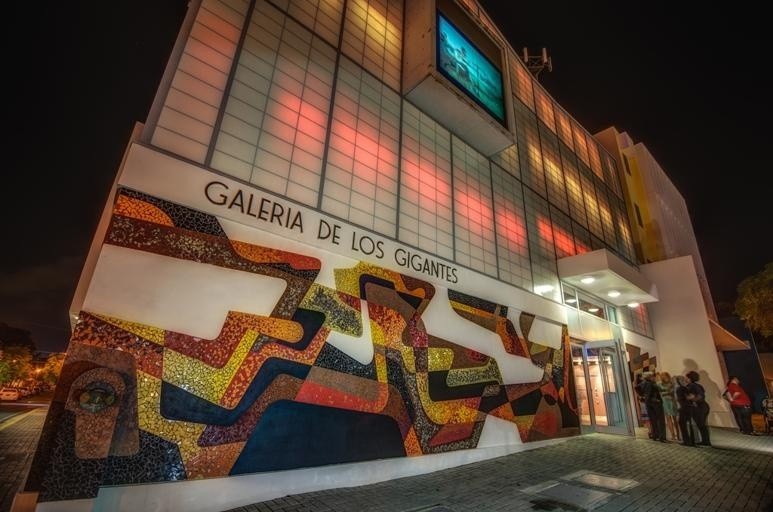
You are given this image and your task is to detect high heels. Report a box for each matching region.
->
[648,432,713,447]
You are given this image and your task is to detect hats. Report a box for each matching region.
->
[686,372,699,381]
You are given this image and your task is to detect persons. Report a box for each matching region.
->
[633,371,712,446]
[723,376,759,436]
